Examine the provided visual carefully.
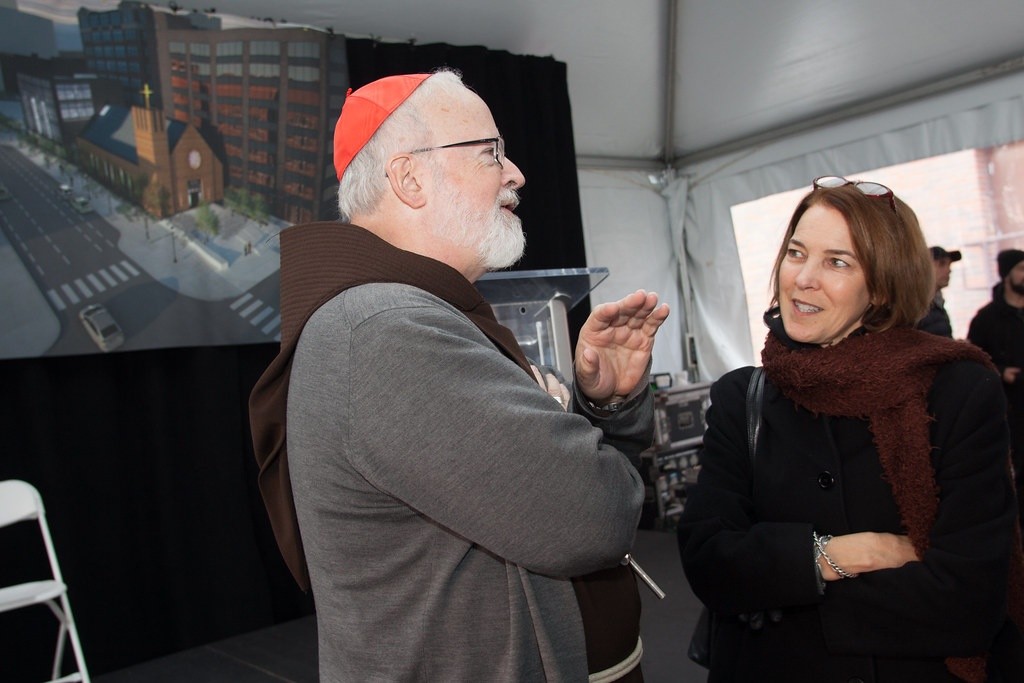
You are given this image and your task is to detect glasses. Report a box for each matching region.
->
[814,175,898,217]
[385,136,505,177]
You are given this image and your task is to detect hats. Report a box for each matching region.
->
[333,74,432,181]
[930,247,962,261]
[997,250,1024,279]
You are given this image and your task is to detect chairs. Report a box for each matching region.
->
[0,480,92,683]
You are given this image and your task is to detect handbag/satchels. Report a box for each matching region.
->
[687,368,765,669]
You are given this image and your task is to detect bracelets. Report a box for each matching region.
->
[812,531,858,583]
[589,399,624,412]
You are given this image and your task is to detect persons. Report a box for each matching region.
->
[248,68,670,682]
[677,176,1018,683]
[916,246,962,340]
[967,248,1024,505]
